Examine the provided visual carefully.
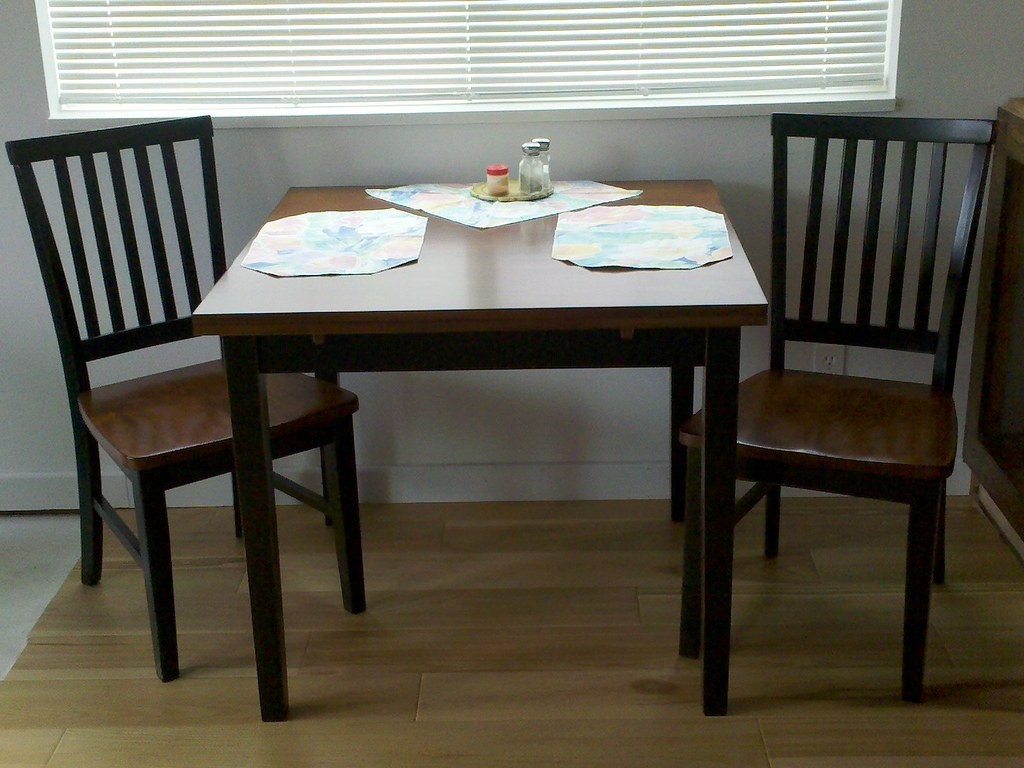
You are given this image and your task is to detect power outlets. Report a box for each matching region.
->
[814,344,845,372]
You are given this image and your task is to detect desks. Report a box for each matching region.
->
[181,171,768,728]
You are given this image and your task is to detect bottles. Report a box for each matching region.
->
[532,138,551,192]
[486,163,509,197]
[518,142,543,194]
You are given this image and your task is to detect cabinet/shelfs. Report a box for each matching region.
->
[960,94,1024,569]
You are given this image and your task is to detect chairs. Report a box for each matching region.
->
[648,99,1001,705]
[3,113,403,682]
